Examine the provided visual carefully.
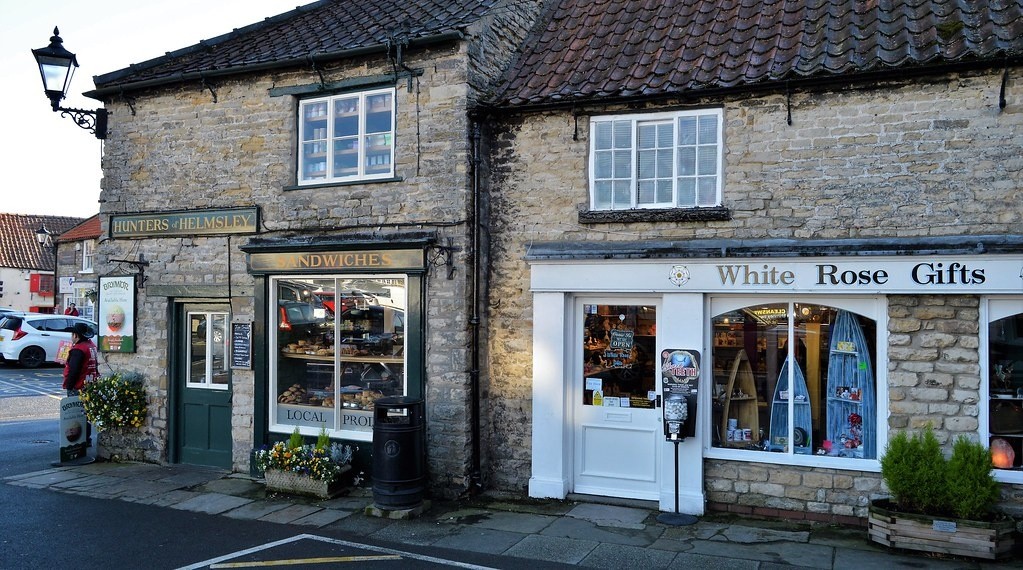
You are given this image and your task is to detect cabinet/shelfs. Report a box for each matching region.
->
[826,308,876,459]
[721,350,759,449]
[768,352,812,456]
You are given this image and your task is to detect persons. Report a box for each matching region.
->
[65,303,79,316]
[62,323,100,447]
[52,303,61,315]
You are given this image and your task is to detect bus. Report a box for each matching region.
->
[306,363,335,390]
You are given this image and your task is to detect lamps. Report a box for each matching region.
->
[35,222,57,249]
[30,26,106,140]
[990,439,1014,470]
[1000,324,1007,342]
[723,316,729,324]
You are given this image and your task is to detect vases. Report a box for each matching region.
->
[265,465,352,499]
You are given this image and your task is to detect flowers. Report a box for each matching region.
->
[250,419,353,485]
[78,370,148,432]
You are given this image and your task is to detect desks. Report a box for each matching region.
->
[280,345,404,412]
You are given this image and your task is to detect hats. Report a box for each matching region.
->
[65,323,94,338]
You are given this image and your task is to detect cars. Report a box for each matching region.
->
[325,305,404,352]
[343,293,369,305]
[301,282,325,292]
[342,338,365,349]
[344,368,353,374]
[362,293,380,305]
[277,280,322,303]
[314,291,348,313]
[197,317,225,343]
[277,299,331,347]
[0,309,99,368]
[373,293,394,305]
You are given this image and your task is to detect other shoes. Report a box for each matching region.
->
[86,438,92,447]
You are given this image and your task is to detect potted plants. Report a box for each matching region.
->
[867,420,1015,563]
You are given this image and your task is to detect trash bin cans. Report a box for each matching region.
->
[371,396,425,511]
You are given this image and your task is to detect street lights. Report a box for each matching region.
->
[36,223,58,309]
